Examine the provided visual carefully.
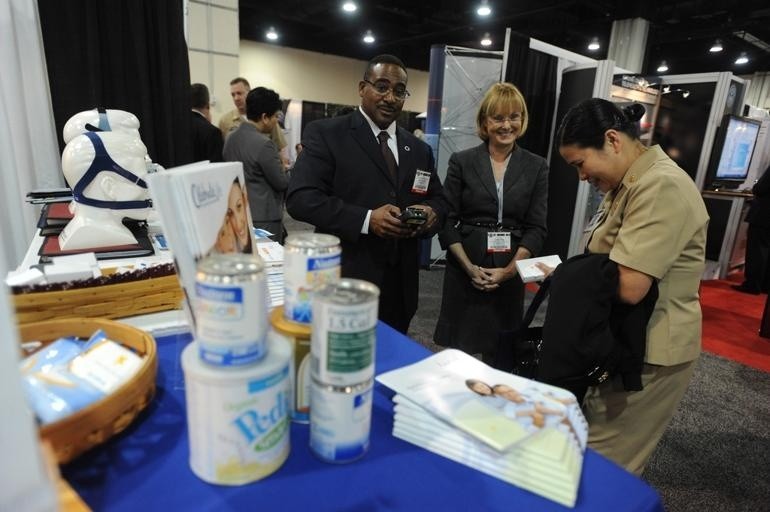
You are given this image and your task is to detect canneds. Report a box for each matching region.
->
[309,376,374,464]
[310,278,381,389]
[193,252,270,370]
[283,233,343,326]
[271,305,311,425]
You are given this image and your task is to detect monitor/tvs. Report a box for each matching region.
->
[704,113,762,190]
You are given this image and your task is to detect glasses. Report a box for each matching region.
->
[364,80,411,101]
[485,113,522,122]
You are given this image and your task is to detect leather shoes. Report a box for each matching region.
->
[730,282,762,296]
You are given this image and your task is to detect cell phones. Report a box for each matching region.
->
[397,207,429,226]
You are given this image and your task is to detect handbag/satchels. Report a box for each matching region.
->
[508,272,558,380]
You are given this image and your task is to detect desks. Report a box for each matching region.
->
[45,289,669,512]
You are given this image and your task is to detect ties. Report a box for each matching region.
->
[377,130,399,190]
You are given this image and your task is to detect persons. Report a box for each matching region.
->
[412,128,427,143]
[220,86,290,247]
[61,108,142,143]
[283,53,453,338]
[294,143,305,155]
[431,79,550,357]
[334,105,356,117]
[535,94,713,477]
[55,131,155,251]
[731,163,770,295]
[189,83,225,163]
[218,77,289,169]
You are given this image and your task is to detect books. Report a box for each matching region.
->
[146,159,285,341]
[376,347,590,511]
[25,187,155,266]
[515,254,563,282]
